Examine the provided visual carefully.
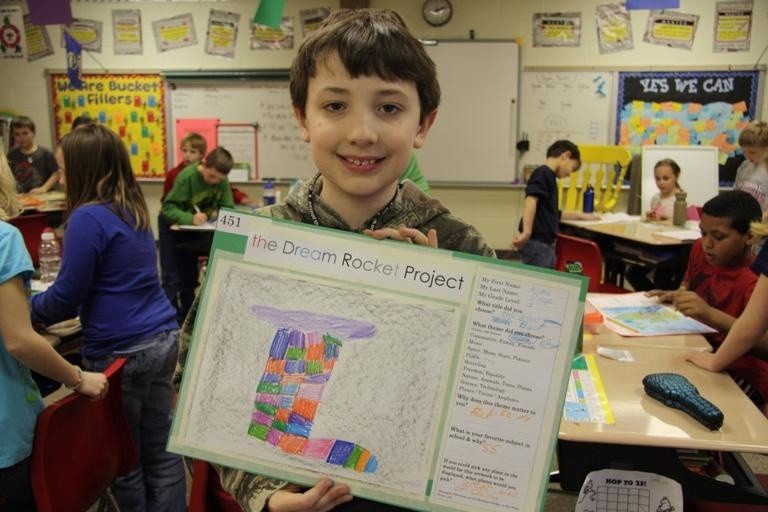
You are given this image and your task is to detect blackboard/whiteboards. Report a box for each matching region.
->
[640,144,719,216]
[516,65,768,189]
[164,38,521,188]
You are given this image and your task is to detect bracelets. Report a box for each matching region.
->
[64,365,84,390]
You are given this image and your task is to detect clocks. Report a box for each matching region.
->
[423,0,453,26]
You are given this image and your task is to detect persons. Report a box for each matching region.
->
[156,146,235,329]
[683,237,768,373]
[511,140,581,270]
[29,122,190,512]
[5,115,61,195]
[169,6,498,512]
[71,115,94,130]
[623,158,689,293]
[159,132,259,209]
[731,120,768,225]
[0,142,110,512]
[644,189,768,414]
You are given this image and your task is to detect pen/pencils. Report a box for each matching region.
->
[193,204,201,214]
[606,317,638,333]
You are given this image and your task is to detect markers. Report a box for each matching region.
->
[280,178,298,181]
[262,177,275,181]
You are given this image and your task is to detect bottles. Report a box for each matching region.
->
[39,233,61,291]
[262,182,276,207]
[582,183,595,212]
[672,192,687,227]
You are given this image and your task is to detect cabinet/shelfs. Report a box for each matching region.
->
[627,156,641,215]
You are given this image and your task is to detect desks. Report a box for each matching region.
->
[7,210,66,265]
[53,331,82,356]
[558,351,768,504]
[171,202,262,256]
[559,218,702,288]
[582,293,713,352]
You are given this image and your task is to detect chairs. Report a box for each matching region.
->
[188,459,242,511]
[556,232,634,293]
[30,357,128,511]
